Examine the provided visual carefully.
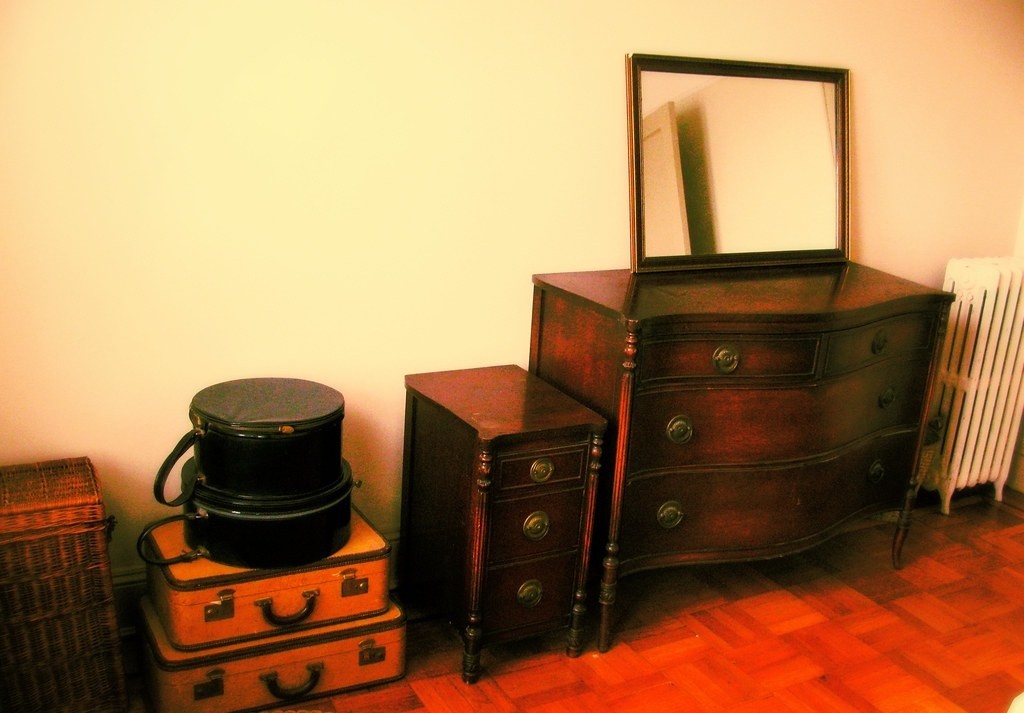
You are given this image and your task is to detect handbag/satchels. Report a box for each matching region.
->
[136,376,354,506]
[135,457,351,571]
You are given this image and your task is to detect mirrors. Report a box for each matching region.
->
[624,52,852,274]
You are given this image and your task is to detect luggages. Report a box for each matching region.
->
[141,506,392,652]
[140,593,408,713]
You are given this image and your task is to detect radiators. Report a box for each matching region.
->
[918,258,1024,515]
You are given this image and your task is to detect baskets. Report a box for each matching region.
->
[0,457,129,713]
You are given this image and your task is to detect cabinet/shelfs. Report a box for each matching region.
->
[404,262,957,684]
